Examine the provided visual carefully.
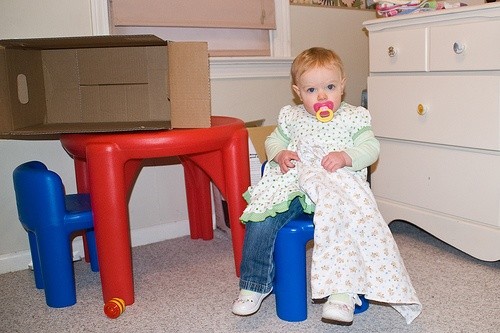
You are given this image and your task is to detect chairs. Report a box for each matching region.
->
[262,160,368,322]
[13,161,99,308]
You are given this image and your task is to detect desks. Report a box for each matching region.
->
[60,116,250,305]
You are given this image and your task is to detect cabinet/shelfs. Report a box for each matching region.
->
[363,2,500,261]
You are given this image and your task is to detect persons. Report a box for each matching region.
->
[232,46,382,325]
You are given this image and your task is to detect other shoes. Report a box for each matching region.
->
[231,286,273,315]
[321,292,363,322]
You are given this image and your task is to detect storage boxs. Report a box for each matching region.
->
[0,34,211,139]
